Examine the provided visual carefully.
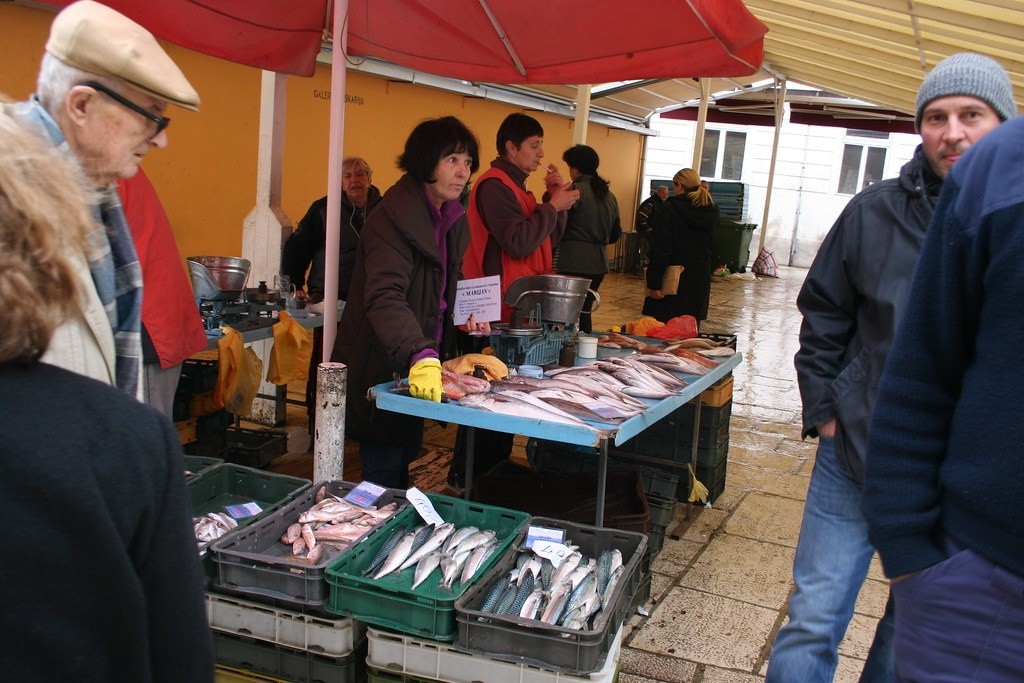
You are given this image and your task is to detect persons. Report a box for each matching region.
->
[0,0,201,403]
[282,157,383,451]
[329,115,492,490]
[638,186,668,280]
[0,91,215,683]
[542,144,622,334]
[115,163,209,422]
[445,113,580,496]
[859,115,1024,683]
[764,51,1017,683]
[701,180,708,191]
[642,168,721,333]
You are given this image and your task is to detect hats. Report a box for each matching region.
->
[44,0,202,112]
[913,52,1016,134]
[563,143,599,169]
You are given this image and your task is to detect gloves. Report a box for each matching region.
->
[409,358,443,402]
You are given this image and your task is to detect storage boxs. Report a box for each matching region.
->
[174,333,740,683]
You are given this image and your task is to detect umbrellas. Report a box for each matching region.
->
[31,0,770,362]
[752,247,779,278]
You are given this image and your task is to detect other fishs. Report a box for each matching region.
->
[192,338,735,638]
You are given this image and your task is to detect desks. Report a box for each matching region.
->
[366,334,742,526]
[202,302,345,432]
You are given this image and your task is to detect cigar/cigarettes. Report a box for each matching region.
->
[539,162,551,172]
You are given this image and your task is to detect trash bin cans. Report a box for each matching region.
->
[712,217,758,273]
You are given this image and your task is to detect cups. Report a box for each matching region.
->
[273,274,296,308]
[578,337,598,360]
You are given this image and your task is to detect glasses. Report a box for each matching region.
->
[82,80,171,141]
